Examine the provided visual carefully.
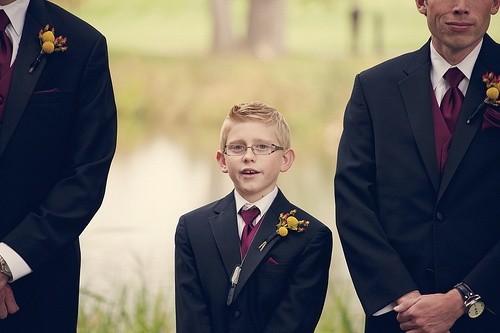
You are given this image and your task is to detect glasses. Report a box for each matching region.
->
[223,143,282,156]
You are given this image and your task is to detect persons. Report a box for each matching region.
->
[335,0,500,333]
[0,1,117,333]
[175,102,332,333]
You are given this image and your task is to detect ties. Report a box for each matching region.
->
[0,10,13,78]
[239,207,260,258]
[441,67,464,130]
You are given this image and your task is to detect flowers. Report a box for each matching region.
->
[465,71,500,123]
[257,210,310,252]
[26,24,68,73]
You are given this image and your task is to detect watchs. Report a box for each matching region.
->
[452,281,485,319]
[0,257,13,277]
